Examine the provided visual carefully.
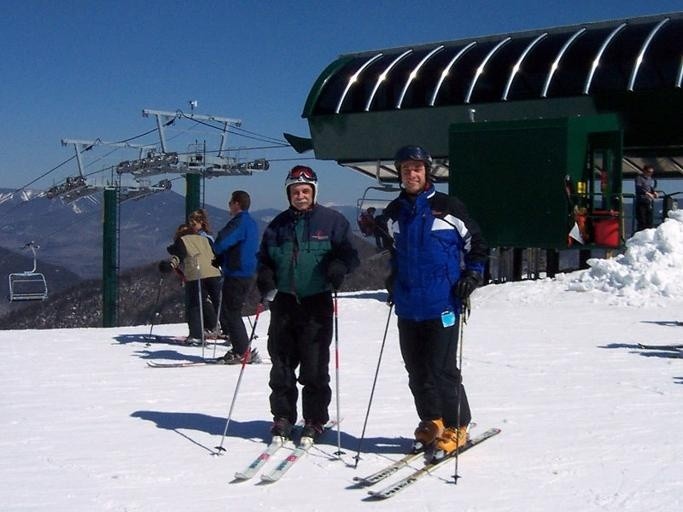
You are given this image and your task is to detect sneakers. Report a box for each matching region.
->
[414,418,445,446]
[270,418,292,437]
[184,327,250,362]
[302,420,326,439]
[434,424,468,453]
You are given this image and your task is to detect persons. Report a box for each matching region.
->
[160,229,223,347]
[376,209,391,249]
[360,146,488,462]
[177,210,222,340]
[257,165,360,447]
[214,191,260,366]
[634,165,659,232]
[361,208,385,252]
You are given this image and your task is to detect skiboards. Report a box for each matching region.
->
[143,335,230,346]
[234,415,343,481]
[147,348,258,368]
[353,423,501,498]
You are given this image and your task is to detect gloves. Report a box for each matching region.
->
[256,271,274,292]
[158,261,172,273]
[325,258,346,290]
[453,268,482,300]
[386,274,393,294]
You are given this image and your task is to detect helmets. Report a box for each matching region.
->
[394,144,432,169]
[283,164,318,207]
